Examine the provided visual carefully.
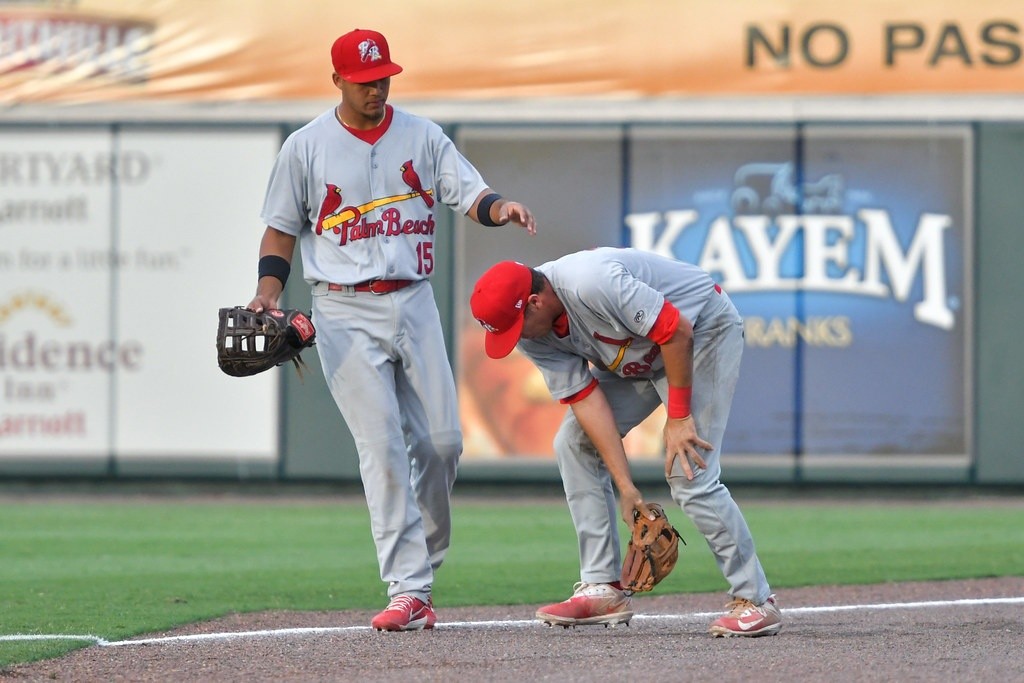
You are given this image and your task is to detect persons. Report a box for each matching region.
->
[245,28,537,631]
[470,246,782,638]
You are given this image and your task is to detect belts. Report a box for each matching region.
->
[714,284,721,294]
[329,279,414,296]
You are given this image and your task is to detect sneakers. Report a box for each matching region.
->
[709,594,783,638]
[372,594,437,631]
[535,582,633,629]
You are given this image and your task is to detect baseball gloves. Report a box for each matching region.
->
[217,307,319,382]
[621,503,686,598]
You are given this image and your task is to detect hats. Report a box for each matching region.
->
[330,29,403,83]
[470,261,533,359]
